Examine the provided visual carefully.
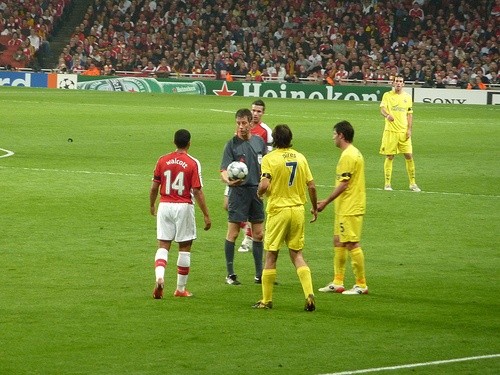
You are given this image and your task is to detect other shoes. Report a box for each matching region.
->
[237,235,254,252]
[384,186,392,191]
[409,184,421,192]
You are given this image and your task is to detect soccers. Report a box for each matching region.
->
[226,161,248,182]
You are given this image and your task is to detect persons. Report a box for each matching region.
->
[150,129,211,299]
[314,120,369,295]
[253,123,318,311]
[379,73,421,192]
[219,108,279,285]
[223,99,273,253]
[0,0,500,91]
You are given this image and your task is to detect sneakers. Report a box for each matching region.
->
[304,294,315,312]
[254,276,278,285]
[152,278,164,298]
[225,273,241,285]
[341,284,368,295]
[174,288,193,296]
[251,300,272,309]
[318,282,344,293]
